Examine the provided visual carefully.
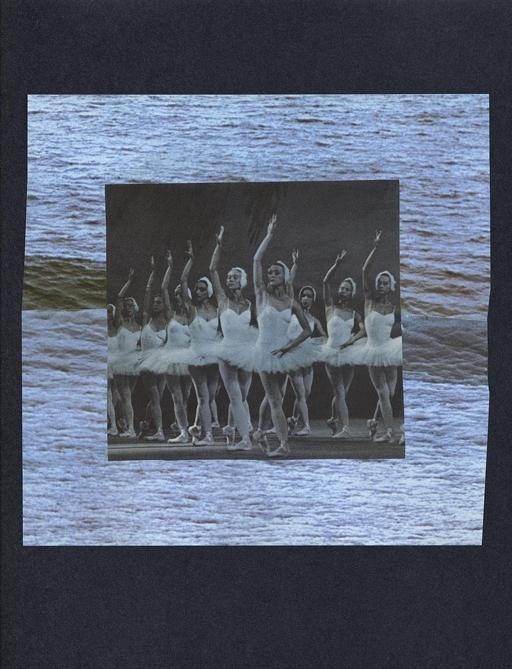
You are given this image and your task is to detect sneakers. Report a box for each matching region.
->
[327,417,404,445]
[223,426,311,457]
[108,427,214,446]
